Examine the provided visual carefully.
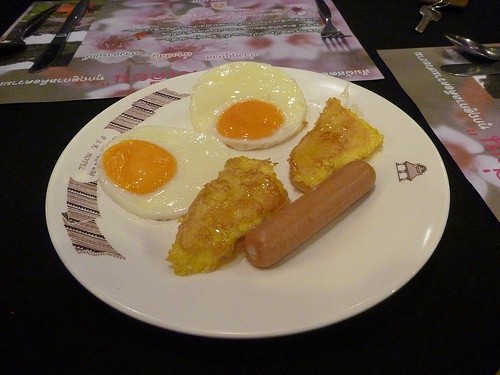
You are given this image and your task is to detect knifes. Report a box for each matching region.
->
[28,0,89,74]
[440,63,500,77]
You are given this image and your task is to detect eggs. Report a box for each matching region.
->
[190,61,307,150]
[95,125,233,221]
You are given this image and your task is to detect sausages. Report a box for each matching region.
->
[243,160,376,267]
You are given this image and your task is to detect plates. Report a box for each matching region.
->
[45,67,450,337]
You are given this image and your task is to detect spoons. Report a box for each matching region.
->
[446,33,500,61]
[0,0,61,49]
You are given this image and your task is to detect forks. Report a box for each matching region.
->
[313,0,351,38]
